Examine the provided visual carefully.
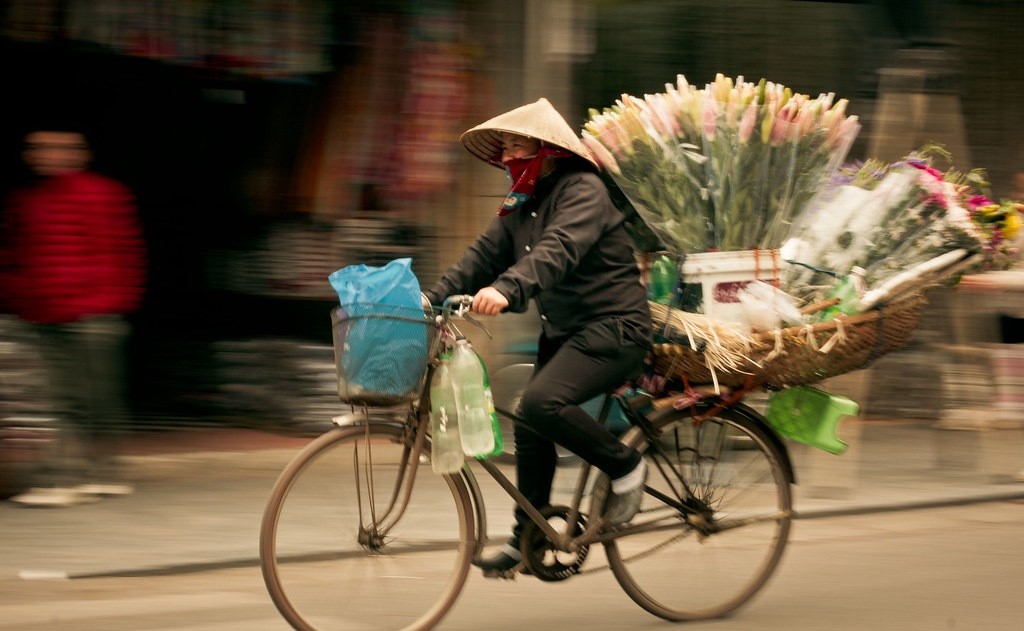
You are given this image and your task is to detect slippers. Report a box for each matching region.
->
[603,462,650,525]
[470,539,532,576]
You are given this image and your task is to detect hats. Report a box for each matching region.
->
[460,97,602,171]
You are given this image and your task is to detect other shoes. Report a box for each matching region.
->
[10,480,134,508]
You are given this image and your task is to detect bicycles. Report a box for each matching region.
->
[258,293,797,631]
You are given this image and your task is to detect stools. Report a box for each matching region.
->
[768,383,860,456]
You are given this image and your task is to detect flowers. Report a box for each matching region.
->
[577,73,1024,323]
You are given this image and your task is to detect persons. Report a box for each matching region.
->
[0,124,142,499]
[417,96,651,572]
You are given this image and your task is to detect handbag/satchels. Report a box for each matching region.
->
[326,258,426,393]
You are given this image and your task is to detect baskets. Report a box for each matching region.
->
[328,303,437,407]
[651,297,926,388]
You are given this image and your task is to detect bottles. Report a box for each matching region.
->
[450,340,494,457]
[814,266,868,345]
[651,254,679,343]
[467,343,504,462]
[429,352,464,474]
